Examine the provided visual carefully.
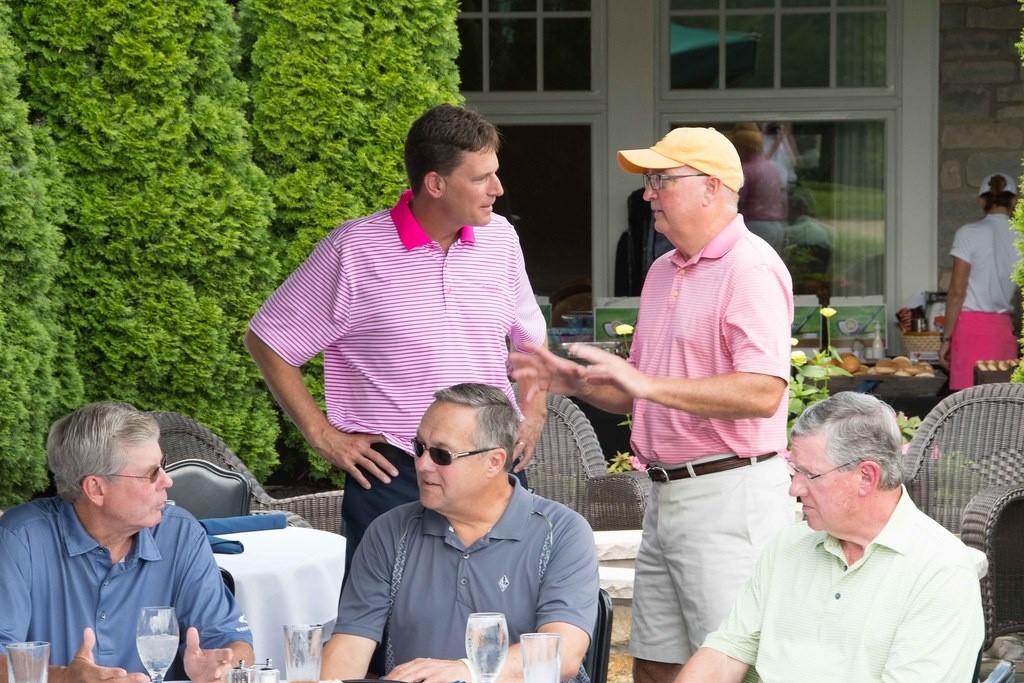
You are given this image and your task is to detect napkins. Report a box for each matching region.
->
[199,512,287,535]
[207,535,244,555]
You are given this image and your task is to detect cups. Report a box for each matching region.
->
[520,633,561,683]
[283,624,323,683]
[6,641,50,683]
[865,347,872,358]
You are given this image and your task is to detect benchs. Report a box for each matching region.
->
[144,380,656,602]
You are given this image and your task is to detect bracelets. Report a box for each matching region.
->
[939,337,950,342]
[460,657,477,683]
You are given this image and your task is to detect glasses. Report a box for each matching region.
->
[787,459,863,485]
[642,172,710,190]
[411,435,502,465]
[79,453,169,484]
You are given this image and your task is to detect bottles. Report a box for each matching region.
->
[230,658,280,683]
[873,323,884,359]
[912,310,921,332]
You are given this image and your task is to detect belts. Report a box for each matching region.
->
[645,451,778,484]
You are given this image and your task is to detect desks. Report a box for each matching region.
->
[213,526,347,681]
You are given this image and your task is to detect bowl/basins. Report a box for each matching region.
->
[561,315,594,328]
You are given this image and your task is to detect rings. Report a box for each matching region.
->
[516,441,523,444]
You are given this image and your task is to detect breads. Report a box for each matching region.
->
[807,352,935,378]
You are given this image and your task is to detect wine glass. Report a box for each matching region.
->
[465,613,509,683]
[136,606,180,683]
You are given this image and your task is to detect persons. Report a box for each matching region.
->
[937,172,1023,390]
[313,383,597,683]
[246,106,550,548]
[670,389,990,683]
[724,122,837,293]
[506,126,798,683]
[0,398,259,683]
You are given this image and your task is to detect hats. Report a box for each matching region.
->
[618,126,744,194]
[979,174,1018,197]
[726,122,765,146]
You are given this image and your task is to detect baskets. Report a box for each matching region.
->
[896,321,945,355]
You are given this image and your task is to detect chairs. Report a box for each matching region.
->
[583,587,613,683]
[162,457,252,517]
[899,382,1024,651]
